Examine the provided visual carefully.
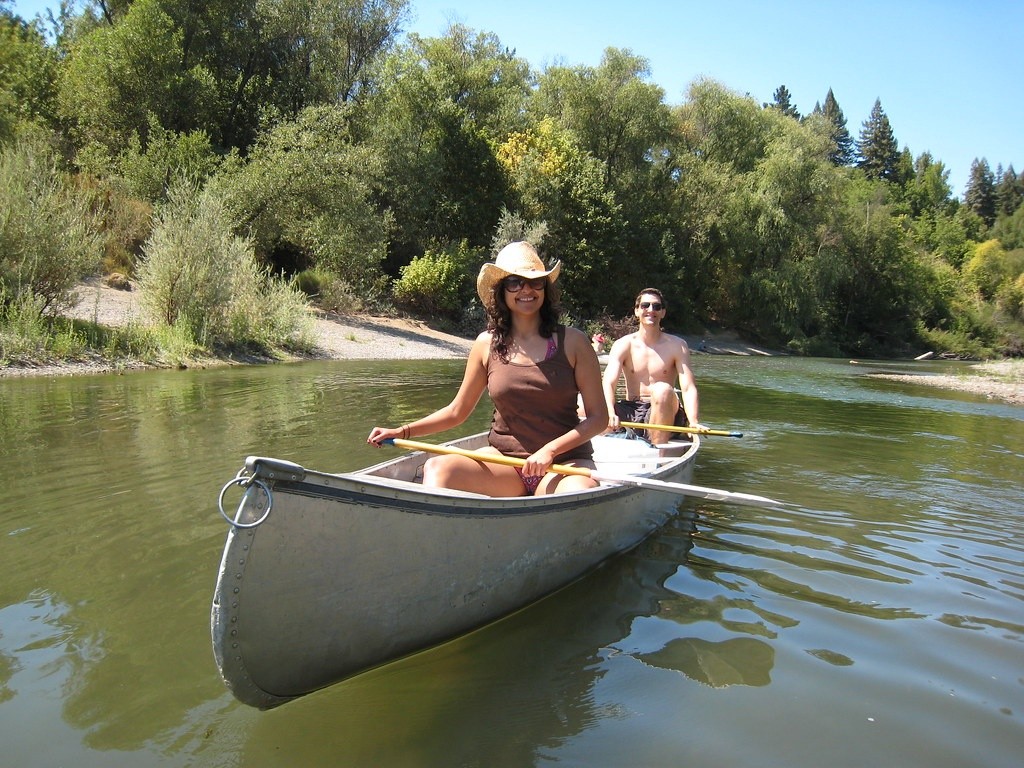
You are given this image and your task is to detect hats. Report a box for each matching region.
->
[477,241,560,309]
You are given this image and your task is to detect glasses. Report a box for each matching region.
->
[502,276,546,292]
[641,302,662,311]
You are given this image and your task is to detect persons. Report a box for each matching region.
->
[366,242,609,498]
[577,287,710,443]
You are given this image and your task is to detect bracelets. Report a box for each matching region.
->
[402,424,410,439]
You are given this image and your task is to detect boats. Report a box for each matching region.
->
[206,379,703,712]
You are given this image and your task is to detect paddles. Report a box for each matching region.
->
[617,422,744,439]
[379,435,788,510]
